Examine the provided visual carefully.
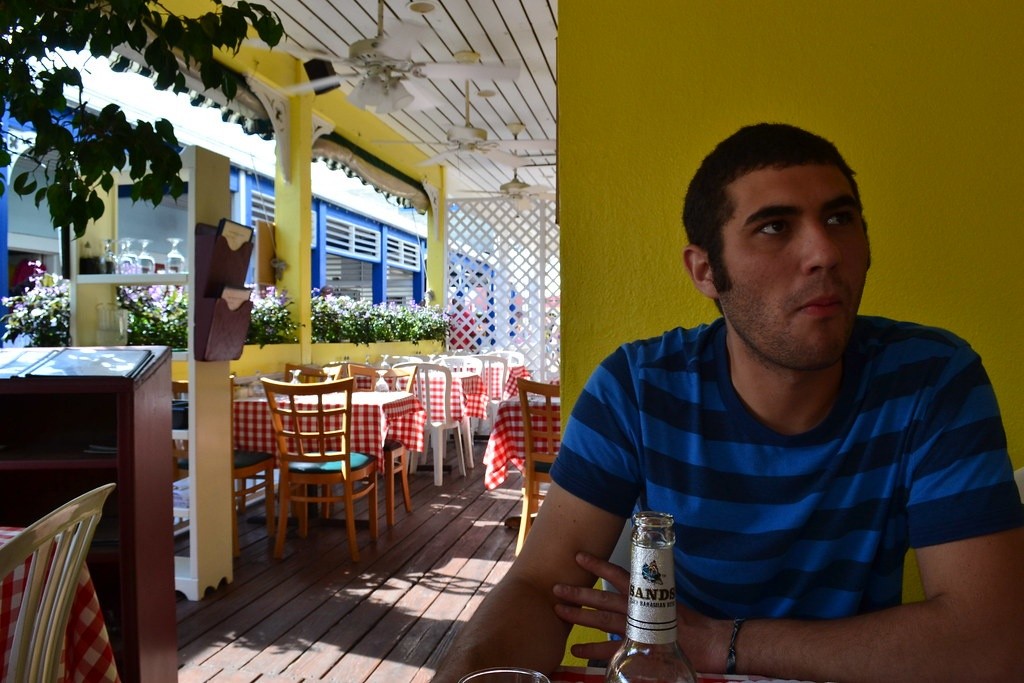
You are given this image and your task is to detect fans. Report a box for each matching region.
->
[231,0,521,114]
[457,122,557,209]
[373,51,557,168]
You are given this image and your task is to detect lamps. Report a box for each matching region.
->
[345,67,415,118]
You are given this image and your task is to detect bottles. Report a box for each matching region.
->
[605,511,697,682]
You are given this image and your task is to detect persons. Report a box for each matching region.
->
[512,311,532,343]
[425,122,1024,683]
[545,312,560,362]
[321,285,336,300]
[448,296,474,350]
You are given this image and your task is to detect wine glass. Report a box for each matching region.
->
[288,369,300,384]
[374,370,389,394]
[325,370,333,382]
[165,237,185,274]
[98,237,155,274]
[364,354,448,370]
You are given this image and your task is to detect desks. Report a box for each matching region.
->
[392,369,479,476]
[451,361,527,443]
[489,392,563,529]
[234,386,418,528]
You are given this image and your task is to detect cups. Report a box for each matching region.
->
[459,666,549,683]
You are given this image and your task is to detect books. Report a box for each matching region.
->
[83,442,118,455]
[0,348,155,378]
[216,217,254,251]
[219,285,252,310]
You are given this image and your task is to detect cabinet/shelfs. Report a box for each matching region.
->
[0,346,179,683]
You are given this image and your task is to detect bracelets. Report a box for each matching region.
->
[726,616,745,676]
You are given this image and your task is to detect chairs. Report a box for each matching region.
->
[493,351,525,364]
[393,360,468,483]
[168,374,277,557]
[440,355,482,469]
[346,362,419,528]
[475,353,508,440]
[517,379,561,556]
[256,374,387,558]
[0,479,120,683]
[281,361,343,388]
